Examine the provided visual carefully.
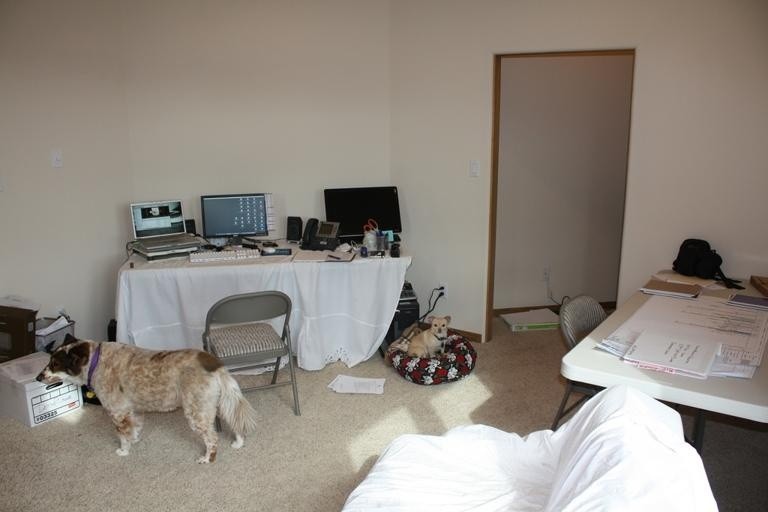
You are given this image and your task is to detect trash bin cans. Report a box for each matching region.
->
[35,316,76,353]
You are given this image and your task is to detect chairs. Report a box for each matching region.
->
[551,293,608,431]
[202,289,304,415]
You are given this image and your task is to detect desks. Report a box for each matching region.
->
[550,268,768,462]
[114,242,413,375]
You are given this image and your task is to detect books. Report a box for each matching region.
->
[291,249,356,263]
[498,307,561,333]
[666,273,718,287]
[621,329,721,381]
[725,294,768,311]
[642,278,702,302]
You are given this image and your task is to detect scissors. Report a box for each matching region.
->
[363,219,379,234]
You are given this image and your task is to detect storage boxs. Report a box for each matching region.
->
[1,351,84,428]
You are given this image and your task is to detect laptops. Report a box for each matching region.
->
[129,199,202,249]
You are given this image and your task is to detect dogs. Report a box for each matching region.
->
[407,315,452,359]
[35,332,257,465]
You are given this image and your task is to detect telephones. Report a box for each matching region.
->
[299,218,341,251]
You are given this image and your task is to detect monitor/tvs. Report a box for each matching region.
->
[200,192,269,245]
[324,184,403,241]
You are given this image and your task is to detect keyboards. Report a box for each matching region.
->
[190,250,257,262]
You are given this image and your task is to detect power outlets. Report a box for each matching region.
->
[439,281,448,299]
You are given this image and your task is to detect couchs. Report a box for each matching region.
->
[341,384,719,512]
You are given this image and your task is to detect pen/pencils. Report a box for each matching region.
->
[328,254,341,260]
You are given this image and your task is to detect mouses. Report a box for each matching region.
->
[260,247,278,256]
